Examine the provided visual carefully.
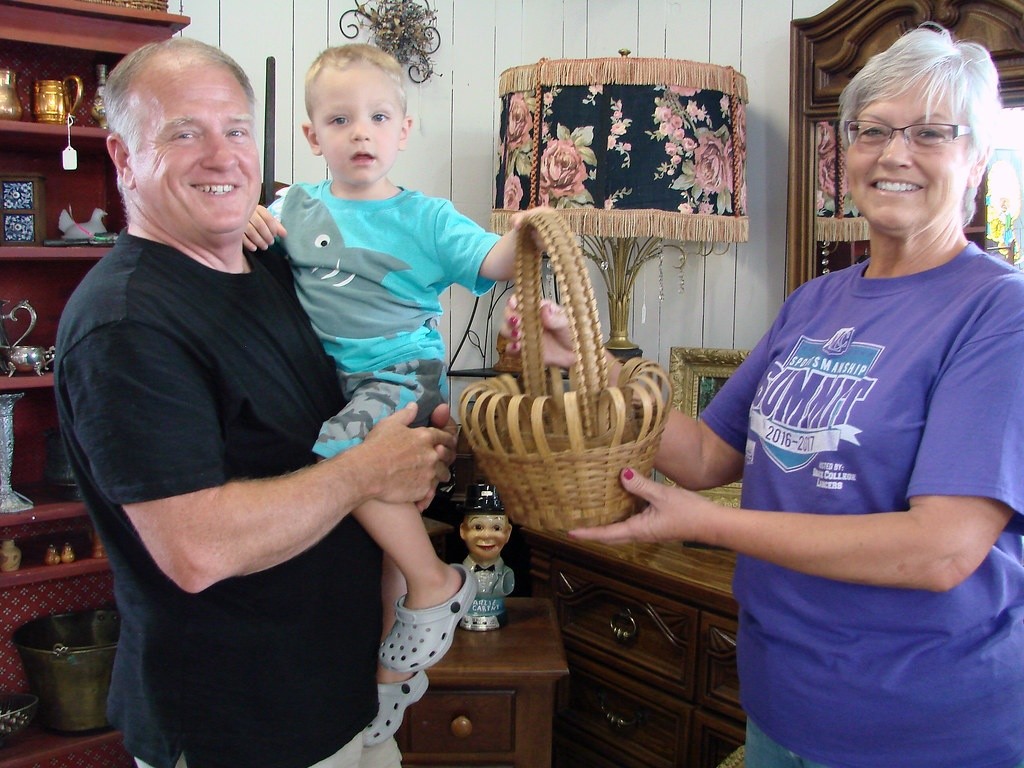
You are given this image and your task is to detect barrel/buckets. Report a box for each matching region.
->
[9,604,124,733]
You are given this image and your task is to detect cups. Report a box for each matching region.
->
[33,75,83,124]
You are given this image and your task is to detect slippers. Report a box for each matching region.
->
[379,563,478,673]
[363,670,430,748]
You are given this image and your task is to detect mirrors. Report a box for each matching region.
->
[780,0,1024,303]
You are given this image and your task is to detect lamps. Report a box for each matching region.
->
[815,121,870,274]
[489,49,751,372]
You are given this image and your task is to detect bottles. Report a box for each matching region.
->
[90,64,109,130]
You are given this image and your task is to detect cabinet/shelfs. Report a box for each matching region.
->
[394,595,570,768]
[0,0,193,768]
[509,527,742,768]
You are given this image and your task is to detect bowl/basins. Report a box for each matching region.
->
[0,693,39,748]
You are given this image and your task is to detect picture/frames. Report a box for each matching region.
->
[654,345,758,508]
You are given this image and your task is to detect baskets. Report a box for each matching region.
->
[455,208,677,535]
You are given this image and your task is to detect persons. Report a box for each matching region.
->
[456,482,517,629]
[493,21,1024,768]
[242,43,549,748]
[55,39,456,767]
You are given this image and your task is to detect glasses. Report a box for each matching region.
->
[844,120,973,154]
